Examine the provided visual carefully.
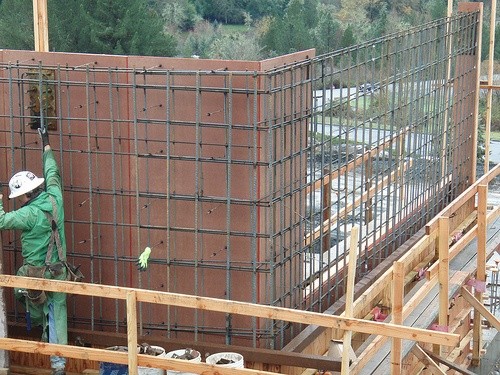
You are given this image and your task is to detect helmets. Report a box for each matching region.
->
[7,171,45,198]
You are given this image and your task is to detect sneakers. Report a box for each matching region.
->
[52,370,65,375]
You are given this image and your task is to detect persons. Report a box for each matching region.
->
[0,127,69,375]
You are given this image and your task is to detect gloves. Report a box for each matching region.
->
[135,246,151,272]
[37,128,51,149]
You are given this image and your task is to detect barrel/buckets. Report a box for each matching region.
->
[206,352,244,369]
[137,346,166,375]
[98,345,129,375]
[165,349,202,375]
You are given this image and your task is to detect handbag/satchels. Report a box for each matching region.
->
[16,262,85,307]
